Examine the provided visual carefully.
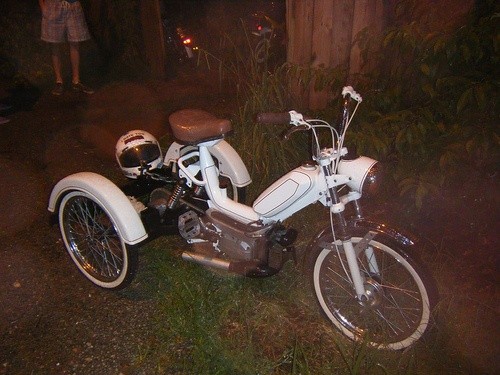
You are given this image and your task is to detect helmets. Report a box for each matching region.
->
[115,130,163,179]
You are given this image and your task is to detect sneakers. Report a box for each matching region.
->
[51,80,66,95]
[71,82,94,94]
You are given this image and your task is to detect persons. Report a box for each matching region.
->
[38,0,97,96]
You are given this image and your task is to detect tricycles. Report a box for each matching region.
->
[45,85,438,354]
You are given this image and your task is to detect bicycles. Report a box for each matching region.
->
[249,1,289,71]
[161,15,196,64]
